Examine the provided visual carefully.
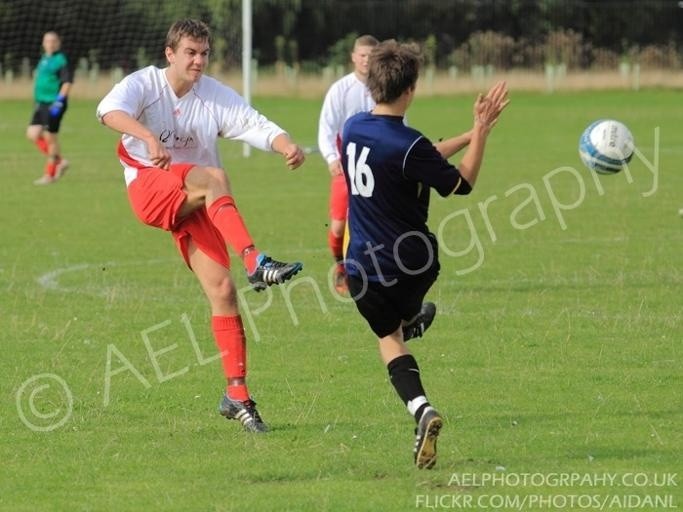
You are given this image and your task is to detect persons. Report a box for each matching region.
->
[26,30,73,185]
[95,18,305,433]
[339,39,510,472]
[317,33,408,293]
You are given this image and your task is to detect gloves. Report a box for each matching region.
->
[49,95,67,117]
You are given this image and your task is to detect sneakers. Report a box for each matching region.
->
[33,158,70,185]
[413,406,444,470]
[247,254,303,293]
[219,391,269,433]
[402,303,436,342]
[335,266,347,293]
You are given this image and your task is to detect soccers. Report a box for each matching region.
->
[577,119,635,176]
